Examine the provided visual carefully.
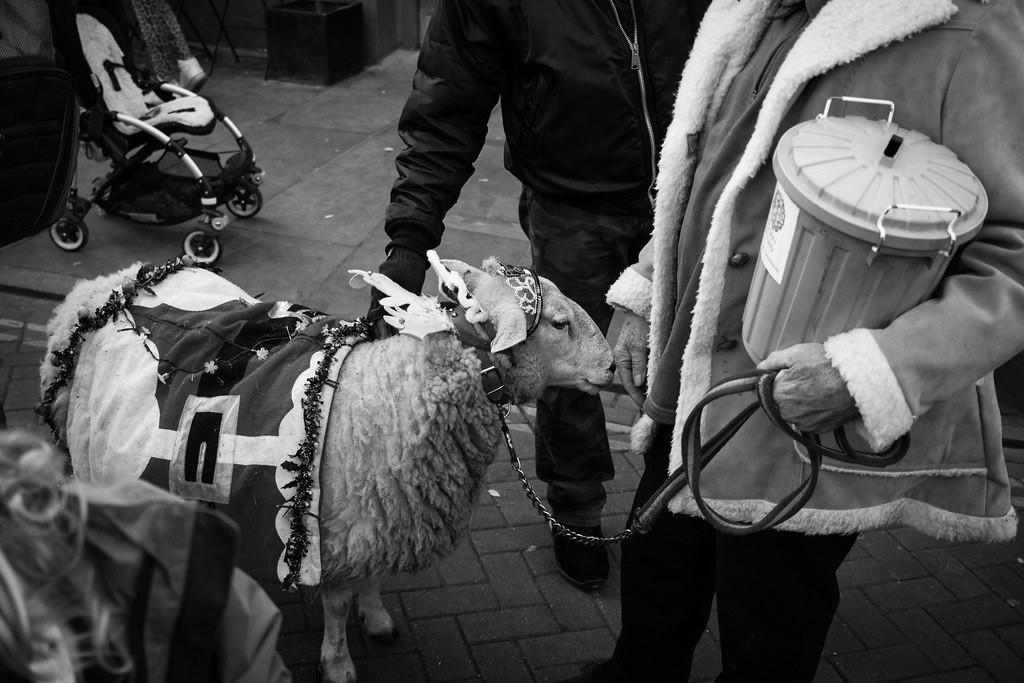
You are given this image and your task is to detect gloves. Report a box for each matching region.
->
[366,247,427,341]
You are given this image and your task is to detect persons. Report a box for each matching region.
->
[582,0,1024,683]
[366,0,713,589]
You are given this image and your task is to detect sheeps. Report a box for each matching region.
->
[41,260,616,683]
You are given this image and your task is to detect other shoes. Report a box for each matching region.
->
[550,515,609,589]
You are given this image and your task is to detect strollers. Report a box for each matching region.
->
[51,0,265,264]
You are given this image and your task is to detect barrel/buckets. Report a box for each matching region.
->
[744,95,992,375]
